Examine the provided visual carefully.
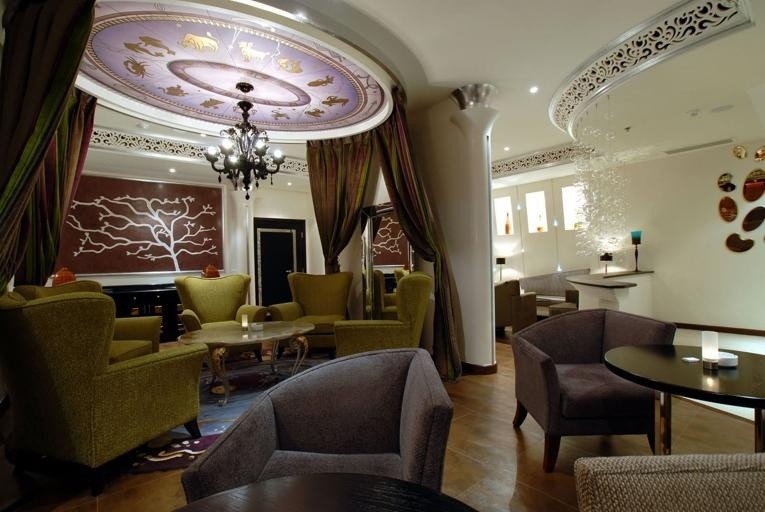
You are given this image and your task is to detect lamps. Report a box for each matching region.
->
[496,258,505,282]
[599,253,613,274]
[205,80,285,199]
[242,313,249,332]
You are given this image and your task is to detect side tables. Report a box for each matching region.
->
[176,322,316,395]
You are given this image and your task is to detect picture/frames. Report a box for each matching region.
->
[47,170,227,278]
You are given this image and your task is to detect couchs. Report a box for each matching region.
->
[495,277,536,336]
[179,347,452,500]
[511,306,676,473]
[519,268,588,316]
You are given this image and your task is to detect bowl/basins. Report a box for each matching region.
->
[249,322,263,331]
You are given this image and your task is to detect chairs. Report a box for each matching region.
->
[372,269,411,320]
[175,274,269,361]
[572,455,765,512]
[334,272,435,358]
[1,279,209,495]
[270,272,353,359]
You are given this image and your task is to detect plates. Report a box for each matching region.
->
[682,356,700,363]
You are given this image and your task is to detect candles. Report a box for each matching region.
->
[630,229,642,245]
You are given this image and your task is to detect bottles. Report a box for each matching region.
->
[52,267,75,286]
[537,213,544,231]
[572,208,583,230]
[504,212,512,234]
[201,263,220,278]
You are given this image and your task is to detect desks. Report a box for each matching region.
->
[603,343,765,453]
[566,270,655,317]
[173,473,479,512]
